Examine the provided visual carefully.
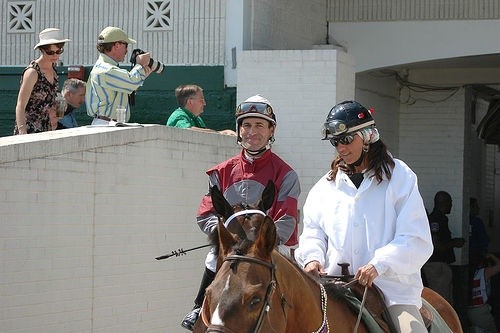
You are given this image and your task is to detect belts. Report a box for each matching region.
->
[95,114,117,122]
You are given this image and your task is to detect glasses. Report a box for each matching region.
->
[43,46,63,56]
[236,101,276,121]
[122,42,128,49]
[329,132,359,147]
[189,95,205,103]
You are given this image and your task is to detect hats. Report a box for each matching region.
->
[34,28,72,50]
[97,26,136,45]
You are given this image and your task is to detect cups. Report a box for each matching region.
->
[116,108,126,124]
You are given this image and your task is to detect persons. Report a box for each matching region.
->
[181,93,302,330]
[469,197,490,252]
[466,240,500,333]
[55,78,86,129]
[12,28,72,135]
[167,84,236,135]
[85,26,153,126]
[293,101,434,333]
[424,190,464,308]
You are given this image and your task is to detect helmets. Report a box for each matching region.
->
[321,100,376,140]
[235,94,276,127]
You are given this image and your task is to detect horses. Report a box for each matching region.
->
[201,214,464,333]
[192,178,279,333]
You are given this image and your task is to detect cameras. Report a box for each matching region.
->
[129,49,165,75]
